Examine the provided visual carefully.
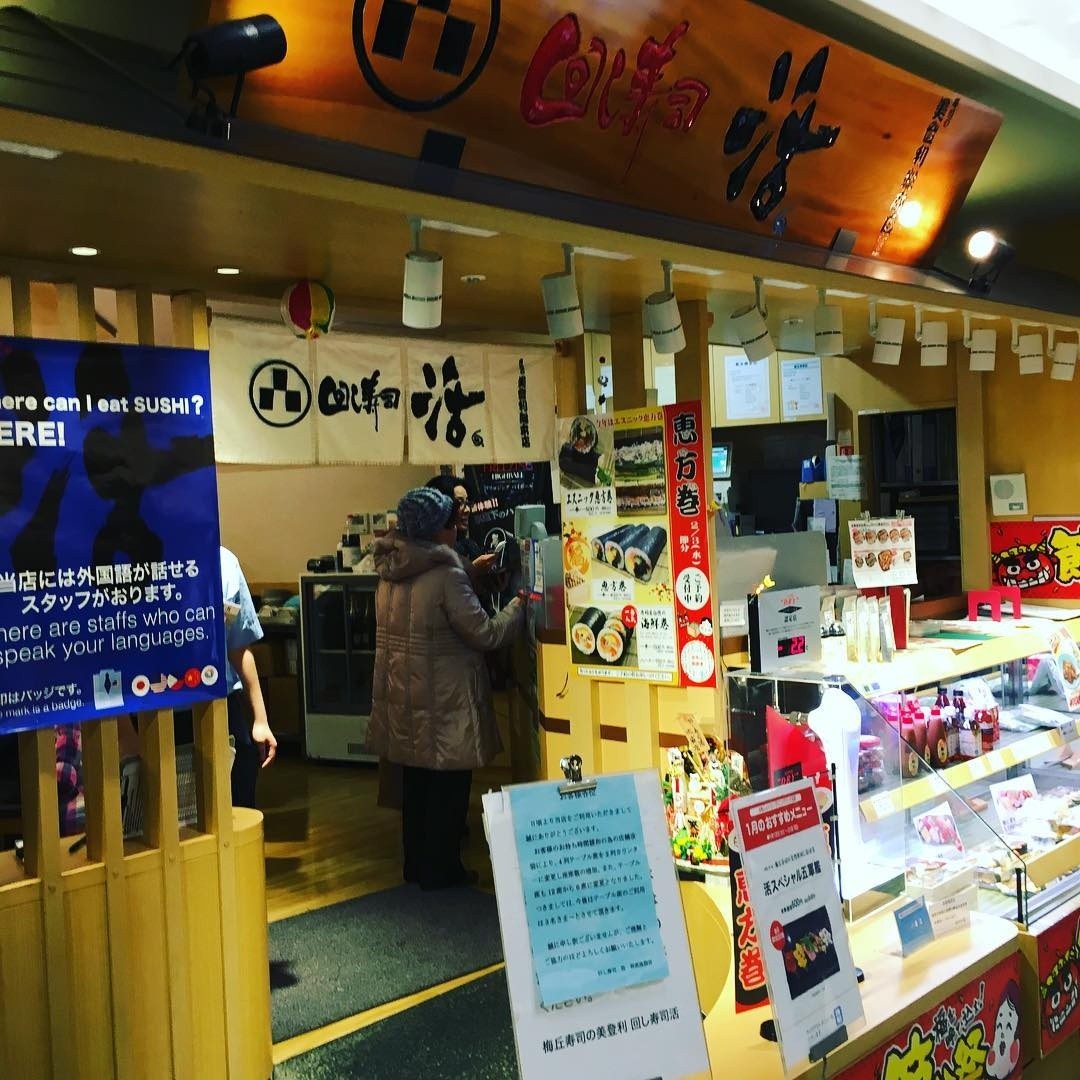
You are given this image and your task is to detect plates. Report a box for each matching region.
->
[816,786,834,814]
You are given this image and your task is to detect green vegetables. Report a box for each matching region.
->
[661,781,712,862]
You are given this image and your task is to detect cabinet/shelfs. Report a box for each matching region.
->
[726,611,1080,930]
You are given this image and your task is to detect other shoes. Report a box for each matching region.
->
[441,871,478,888]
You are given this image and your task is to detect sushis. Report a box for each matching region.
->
[591,524,668,581]
[571,606,634,664]
[558,417,605,486]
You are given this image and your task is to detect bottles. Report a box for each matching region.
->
[856,687,1002,794]
[341,514,361,573]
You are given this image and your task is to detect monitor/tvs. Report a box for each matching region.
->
[712,441,734,481]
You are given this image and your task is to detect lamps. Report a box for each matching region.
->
[1047,327,1078,380]
[644,260,687,356]
[816,288,843,356]
[402,215,443,329]
[542,242,582,342]
[963,312,996,372]
[177,14,286,137]
[732,277,775,363]
[967,229,1016,295]
[870,300,906,366]
[915,305,948,367]
[1011,321,1043,375]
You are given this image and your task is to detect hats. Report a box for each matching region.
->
[397,487,453,540]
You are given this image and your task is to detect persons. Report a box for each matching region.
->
[220,545,290,975]
[55,721,86,837]
[366,475,526,892]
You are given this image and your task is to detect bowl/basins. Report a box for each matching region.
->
[258,605,296,624]
[306,554,335,574]
